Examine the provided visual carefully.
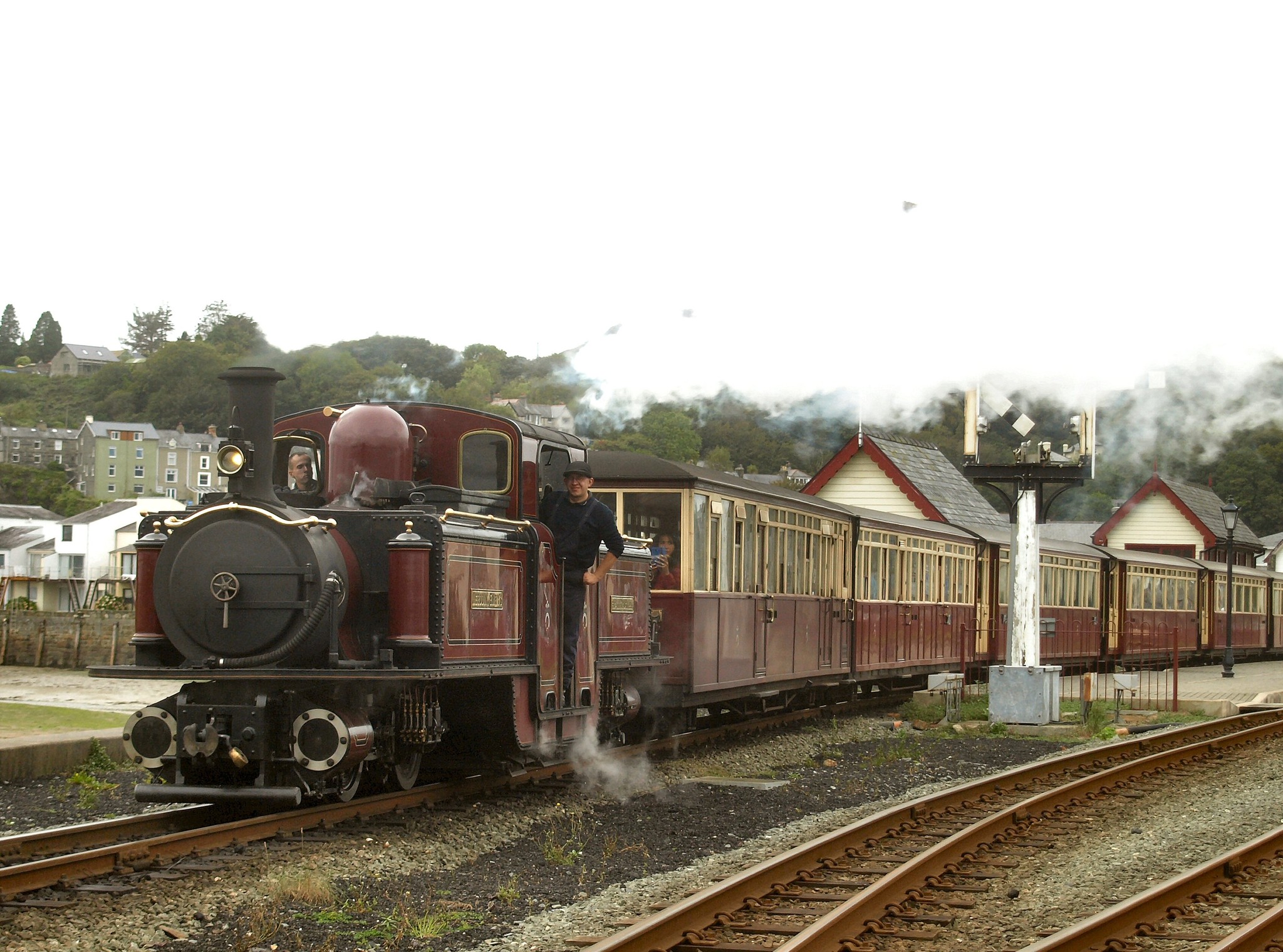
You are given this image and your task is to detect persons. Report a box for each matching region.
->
[650,531,681,590]
[288,452,317,491]
[539,461,625,706]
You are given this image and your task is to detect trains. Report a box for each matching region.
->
[88,367,1282,811]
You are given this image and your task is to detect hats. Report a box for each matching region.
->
[563,461,592,478]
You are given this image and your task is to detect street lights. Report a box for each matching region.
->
[1220,494,1243,678]
